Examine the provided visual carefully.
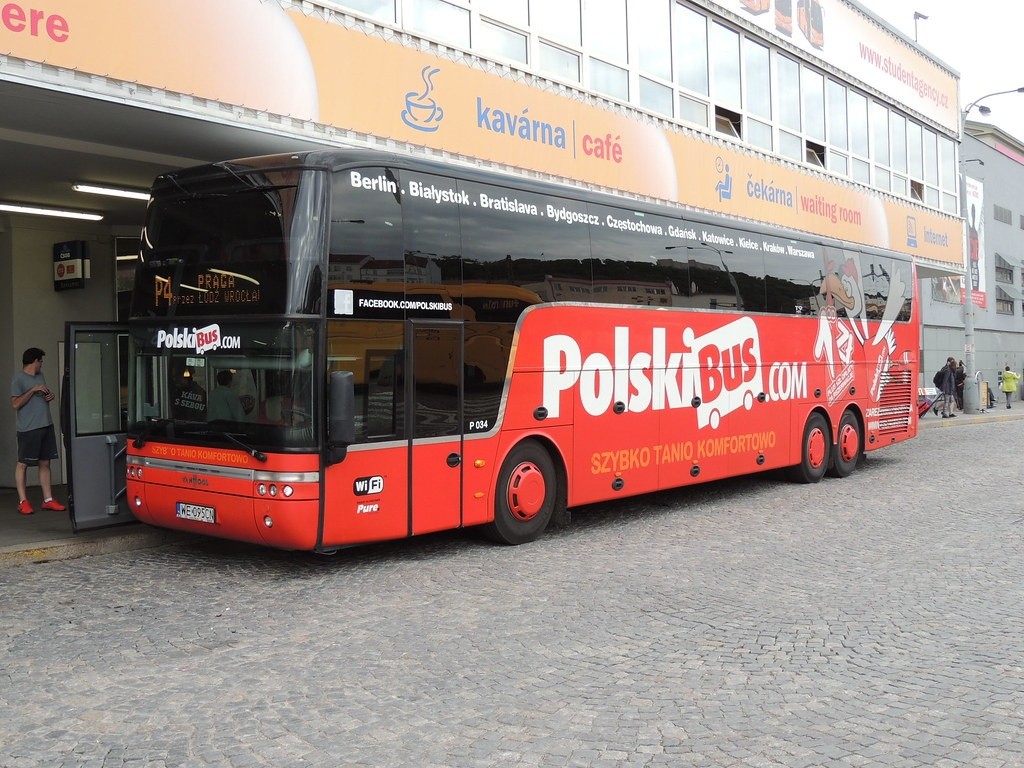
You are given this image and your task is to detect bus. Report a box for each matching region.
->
[125,146,921,555]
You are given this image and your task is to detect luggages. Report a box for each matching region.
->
[918,392,944,418]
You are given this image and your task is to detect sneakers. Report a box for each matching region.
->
[40,498,66,511]
[18,499,34,515]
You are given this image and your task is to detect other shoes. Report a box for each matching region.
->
[933,407,938,416]
[942,415,949,418]
[1007,405,1011,409]
[949,413,957,417]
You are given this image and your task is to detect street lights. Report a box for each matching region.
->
[959,87,1024,413]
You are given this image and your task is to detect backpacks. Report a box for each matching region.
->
[933,365,949,388]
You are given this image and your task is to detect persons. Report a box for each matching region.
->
[367,367,381,394]
[988,388,996,408]
[933,357,967,418]
[207,369,247,422]
[1002,366,1021,409]
[10,347,66,515]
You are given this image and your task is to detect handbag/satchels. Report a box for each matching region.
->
[957,383,964,390]
[999,383,1004,394]
